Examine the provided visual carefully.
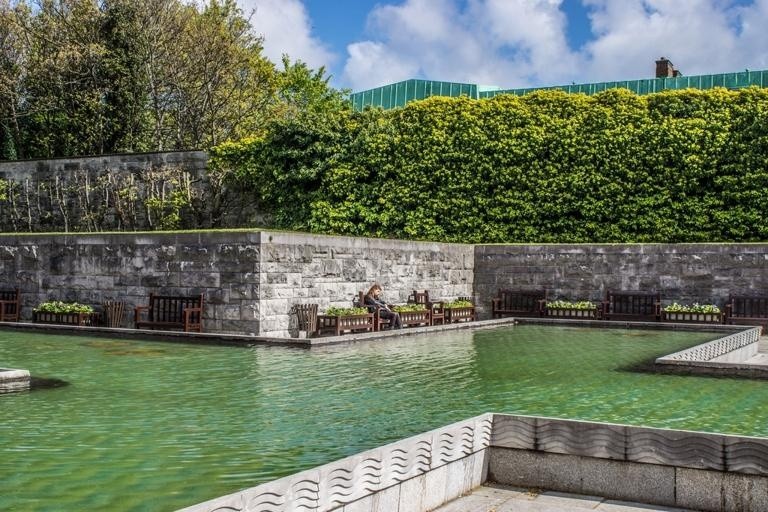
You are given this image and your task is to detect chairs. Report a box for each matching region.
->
[359,291,393,331]
[413,290,444,325]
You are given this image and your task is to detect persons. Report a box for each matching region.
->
[364,285,403,330]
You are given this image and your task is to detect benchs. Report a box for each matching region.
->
[601,291,661,321]
[0,288,21,322]
[134,293,204,332]
[492,288,547,319]
[725,293,768,325]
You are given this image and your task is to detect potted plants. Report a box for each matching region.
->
[435,300,472,323]
[390,303,426,322]
[323,306,368,333]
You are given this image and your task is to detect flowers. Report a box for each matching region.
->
[665,302,719,320]
[548,301,596,316]
[32,300,96,326]
